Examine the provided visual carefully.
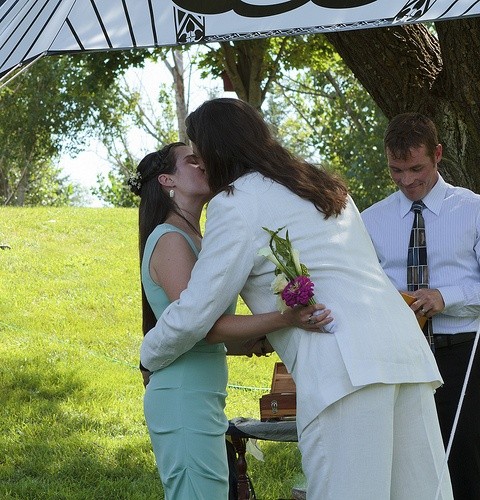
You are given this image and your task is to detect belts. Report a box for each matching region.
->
[434,331,476,348]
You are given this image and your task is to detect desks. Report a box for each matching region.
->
[228,420,301,500]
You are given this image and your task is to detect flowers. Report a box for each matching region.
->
[255,225,339,334]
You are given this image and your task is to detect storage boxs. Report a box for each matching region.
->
[259,360,298,420]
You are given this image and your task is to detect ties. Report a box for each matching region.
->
[406,200,435,352]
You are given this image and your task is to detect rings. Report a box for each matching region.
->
[421,308,427,313]
[308,315,313,324]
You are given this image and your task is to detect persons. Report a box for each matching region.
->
[360,113,480,500]
[135,142,334,500]
[140,98,454,500]
